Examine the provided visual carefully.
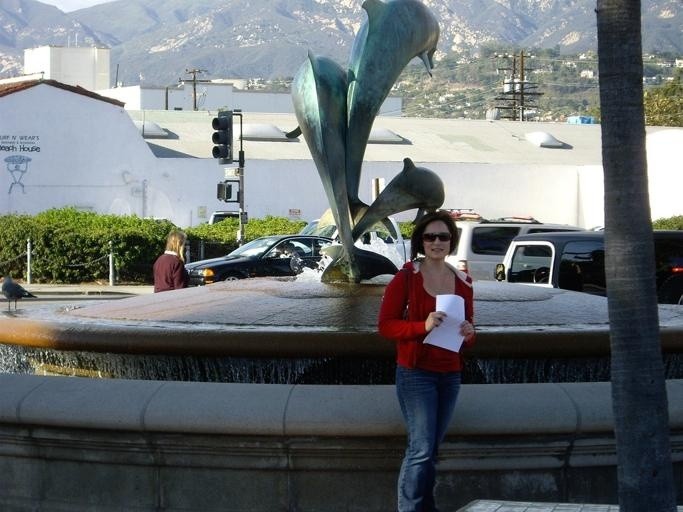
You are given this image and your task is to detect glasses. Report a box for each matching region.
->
[422,232,451,242]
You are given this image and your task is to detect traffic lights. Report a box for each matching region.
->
[212,110,233,164]
[216,181,232,200]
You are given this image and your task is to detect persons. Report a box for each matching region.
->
[275,243,299,258]
[153,230,190,293]
[377,211,477,512]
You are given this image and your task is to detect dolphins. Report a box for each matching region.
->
[320,156,446,260]
[316,1,439,240]
[284,49,399,284]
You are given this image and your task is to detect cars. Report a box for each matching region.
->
[181,234,401,289]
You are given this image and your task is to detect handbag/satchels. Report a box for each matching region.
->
[369,332,396,368]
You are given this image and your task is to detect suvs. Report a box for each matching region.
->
[494,229,683,304]
[444,214,588,281]
